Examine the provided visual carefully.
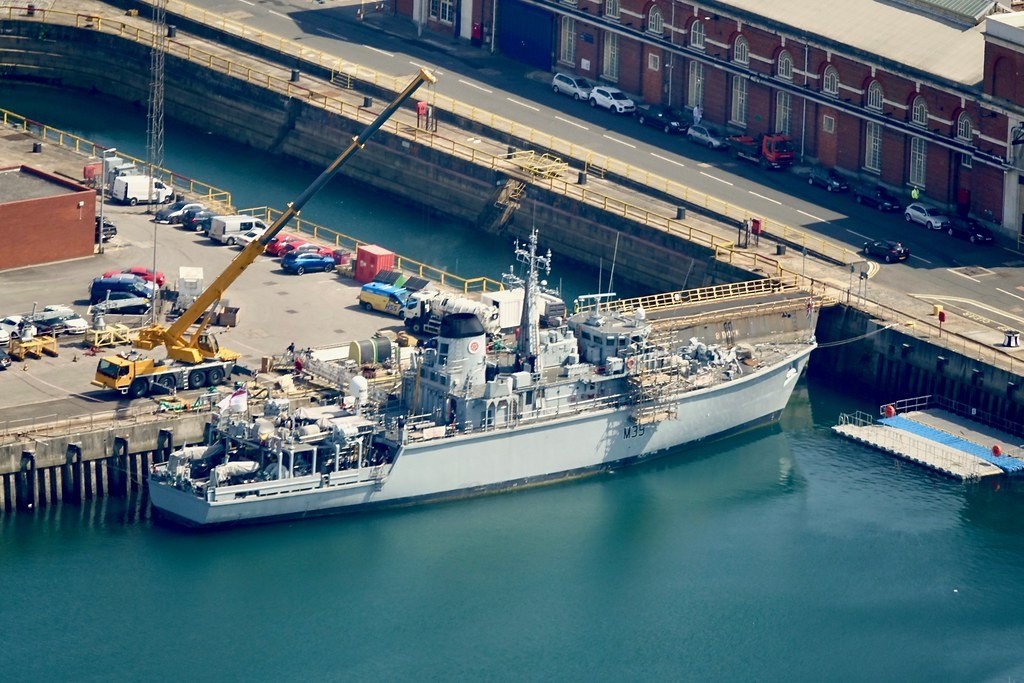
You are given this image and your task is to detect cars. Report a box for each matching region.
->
[281,251,336,276]
[155,199,214,232]
[280,241,334,257]
[0,305,88,368]
[266,234,300,257]
[865,238,911,265]
[237,229,264,248]
[550,71,998,249]
[101,267,166,287]
[93,292,153,314]
[108,274,159,293]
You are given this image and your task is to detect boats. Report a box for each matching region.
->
[146,227,817,532]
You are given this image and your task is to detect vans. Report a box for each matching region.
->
[208,216,269,246]
[113,177,175,208]
[88,278,153,305]
[358,282,410,320]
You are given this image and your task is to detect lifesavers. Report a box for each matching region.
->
[626,358,636,369]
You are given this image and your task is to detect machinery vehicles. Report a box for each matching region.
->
[404,290,500,336]
[90,69,439,399]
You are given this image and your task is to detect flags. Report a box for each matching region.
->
[215,381,247,415]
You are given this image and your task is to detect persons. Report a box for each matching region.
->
[693,104,702,125]
[911,185,920,202]
[286,342,295,356]
[294,357,303,374]
[305,347,315,359]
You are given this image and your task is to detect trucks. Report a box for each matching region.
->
[721,129,796,170]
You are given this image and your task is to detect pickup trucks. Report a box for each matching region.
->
[95,216,118,242]
